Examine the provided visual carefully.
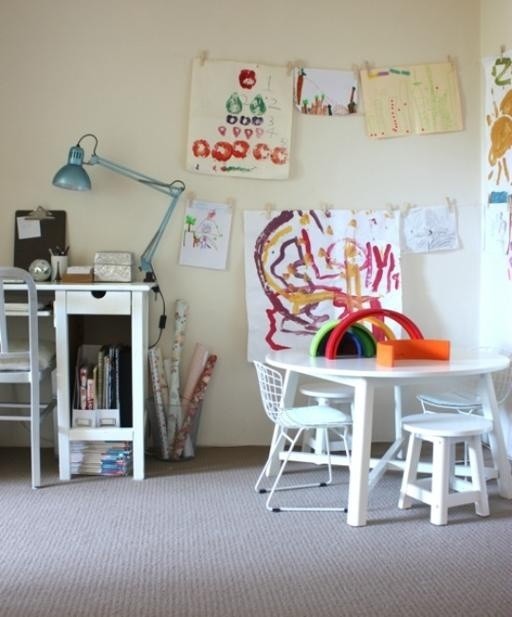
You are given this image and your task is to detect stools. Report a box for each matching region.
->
[297,384,353,457]
[402,414,493,523]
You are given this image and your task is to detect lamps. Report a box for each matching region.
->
[53,132,185,282]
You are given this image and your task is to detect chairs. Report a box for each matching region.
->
[415,347,511,479]
[1,279,61,491]
[252,361,352,514]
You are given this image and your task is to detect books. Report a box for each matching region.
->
[69,343,133,476]
[3,296,53,313]
[0,275,27,284]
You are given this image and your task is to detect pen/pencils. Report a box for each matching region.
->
[49,245,70,256]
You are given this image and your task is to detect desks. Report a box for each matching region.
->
[2,281,157,482]
[269,346,511,527]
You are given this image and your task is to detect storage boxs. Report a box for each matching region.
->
[95,251,134,281]
